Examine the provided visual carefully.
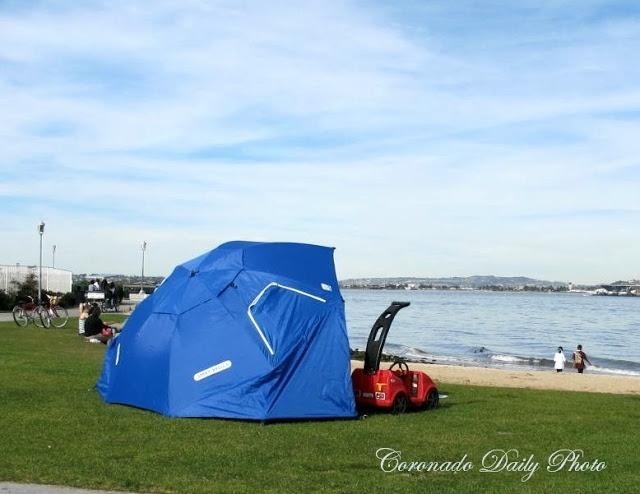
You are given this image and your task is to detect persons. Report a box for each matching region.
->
[78,301,125,343]
[571,344,592,373]
[553,346,566,372]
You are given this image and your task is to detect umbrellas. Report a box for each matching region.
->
[94,240,362,420]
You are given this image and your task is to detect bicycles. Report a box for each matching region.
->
[12,293,46,327]
[38,291,69,330]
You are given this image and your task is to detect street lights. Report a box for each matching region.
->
[37,221,45,304]
[52,244,56,267]
[140,240,147,292]
